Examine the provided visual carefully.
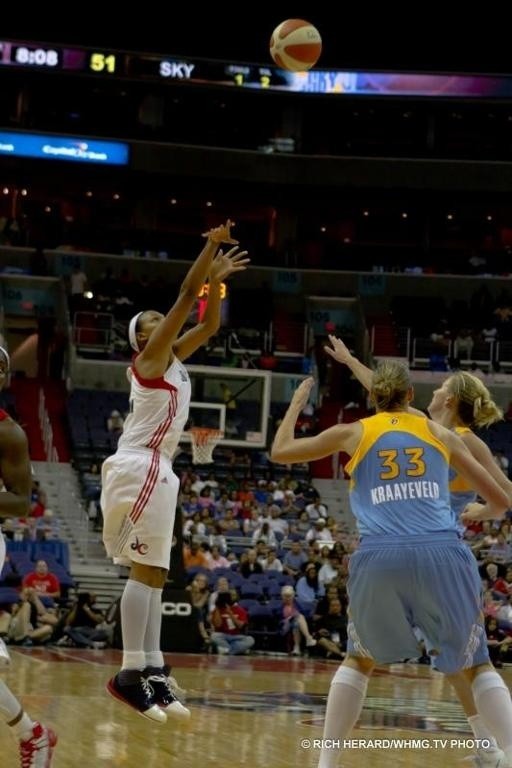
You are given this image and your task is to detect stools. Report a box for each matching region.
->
[0,472,77,614]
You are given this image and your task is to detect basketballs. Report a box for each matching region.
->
[269,19,322,70]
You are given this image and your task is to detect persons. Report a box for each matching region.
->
[99,218,252,723]
[1,348,512,668]
[323,334,512,767]
[0,345,56,767]
[270,359,512,768]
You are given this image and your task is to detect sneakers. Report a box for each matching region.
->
[106,665,191,724]
[473,750,506,768]
[217,646,230,654]
[18,722,58,768]
[306,638,316,647]
[245,650,250,655]
[291,645,300,655]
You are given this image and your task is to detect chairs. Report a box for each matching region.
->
[66,388,351,637]
